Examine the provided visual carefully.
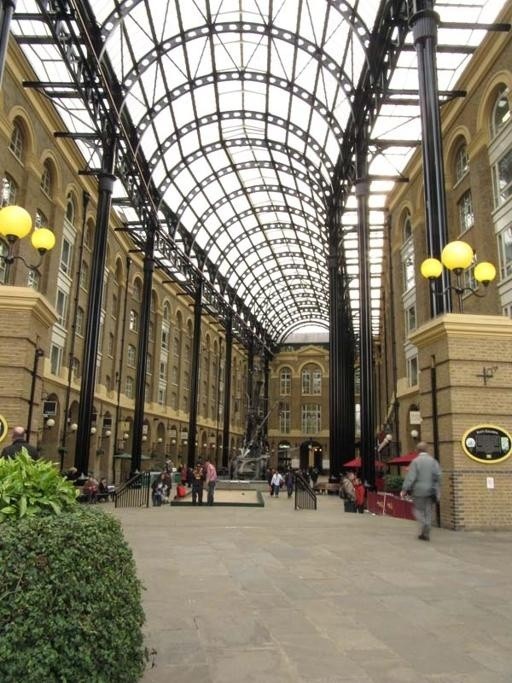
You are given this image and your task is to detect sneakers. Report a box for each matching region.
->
[419,525,431,541]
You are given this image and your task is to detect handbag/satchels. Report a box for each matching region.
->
[431,482,440,502]
[339,487,346,499]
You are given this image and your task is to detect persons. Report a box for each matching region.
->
[151,454,173,507]
[267,464,320,498]
[400,442,441,541]
[0,426,39,461]
[84,476,108,504]
[339,471,369,513]
[180,459,218,506]
